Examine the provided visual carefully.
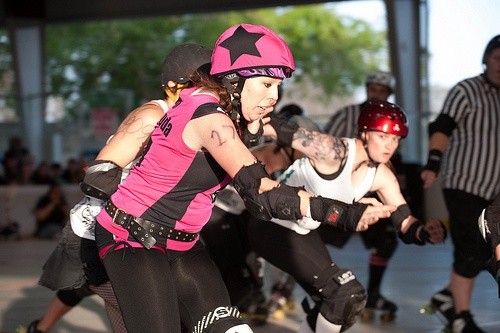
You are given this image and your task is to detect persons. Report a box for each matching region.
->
[26,43,214,332]
[200,70,447,333]
[420,34,500,333]
[94,22,398,333]
[0,137,100,238]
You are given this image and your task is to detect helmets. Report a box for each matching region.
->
[290,115,323,134]
[357,100,409,138]
[159,43,214,84]
[209,24,296,79]
[366,70,397,92]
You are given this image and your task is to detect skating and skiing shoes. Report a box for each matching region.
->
[442,310,486,333]
[15,318,41,333]
[265,284,296,321]
[421,288,454,323]
[360,293,398,322]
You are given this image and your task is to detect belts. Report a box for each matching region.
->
[77,181,198,250]
